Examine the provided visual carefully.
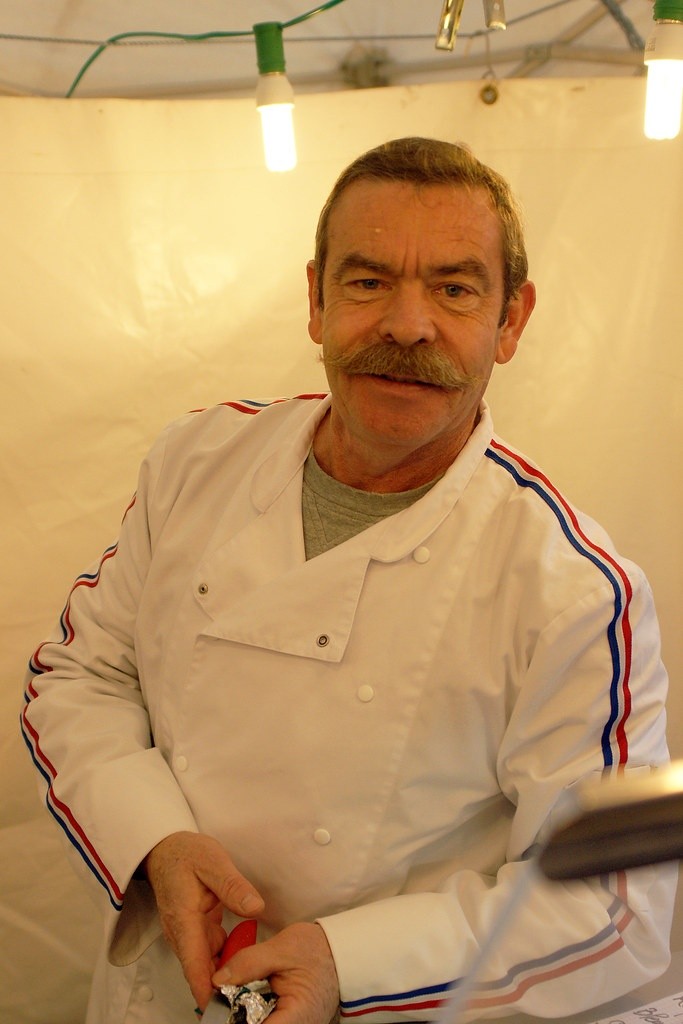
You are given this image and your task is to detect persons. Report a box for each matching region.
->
[17,136,681,1023]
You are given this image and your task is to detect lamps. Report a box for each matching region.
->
[641,0,683,141]
[254,21,304,177]
